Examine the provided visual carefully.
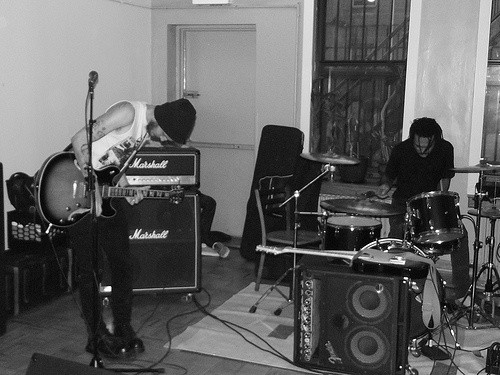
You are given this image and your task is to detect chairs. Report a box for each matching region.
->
[254,187,321,303]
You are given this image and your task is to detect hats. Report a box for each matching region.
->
[154,98,196,144]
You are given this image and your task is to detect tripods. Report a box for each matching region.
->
[418,170,500,346]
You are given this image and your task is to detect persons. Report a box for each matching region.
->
[372,117,457,315]
[70,98,196,362]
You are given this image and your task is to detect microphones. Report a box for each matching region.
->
[89,71,98,84]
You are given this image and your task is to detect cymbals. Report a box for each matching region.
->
[448,164,500,173]
[299,152,360,165]
[467,208,500,221]
[320,198,405,216]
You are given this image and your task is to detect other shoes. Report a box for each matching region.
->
[455,295,480,310]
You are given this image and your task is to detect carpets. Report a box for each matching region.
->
[163,281,500,375]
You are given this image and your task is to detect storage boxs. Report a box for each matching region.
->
[4,209,69,315]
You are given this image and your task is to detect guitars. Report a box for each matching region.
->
[33,152,185,228]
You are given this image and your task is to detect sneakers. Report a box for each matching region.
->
[212,242,229,258]
[201,243,220,256]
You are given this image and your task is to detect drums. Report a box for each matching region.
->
[322,216,382,251]
[358,238,426,257]
[405,191,464,245]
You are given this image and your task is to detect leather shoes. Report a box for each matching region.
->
[115,326,144,352]
[86,333,134,358]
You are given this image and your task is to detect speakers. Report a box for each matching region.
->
[26,353,122,375]
[94,189,203,295]
[295,254,412,375]
